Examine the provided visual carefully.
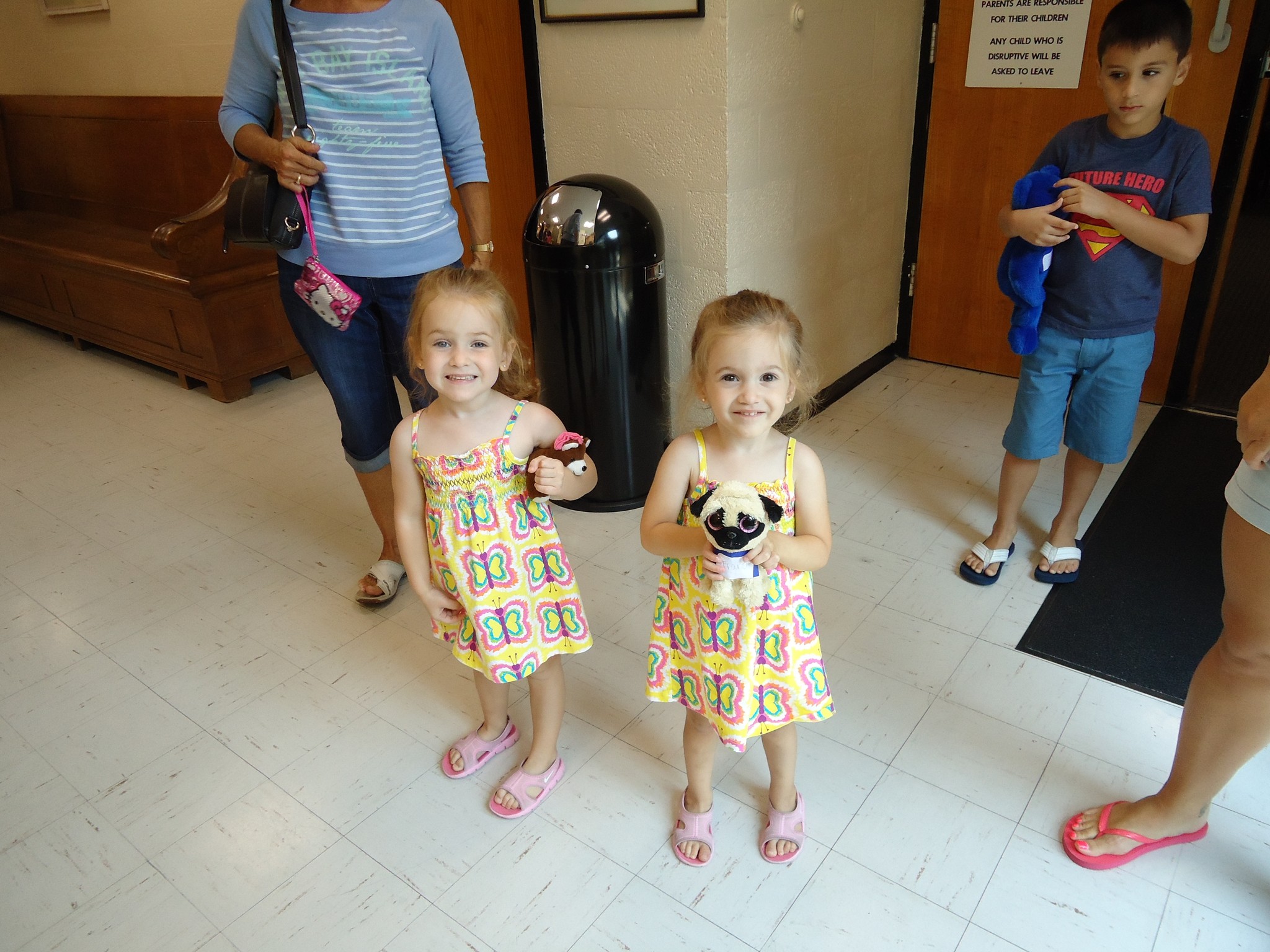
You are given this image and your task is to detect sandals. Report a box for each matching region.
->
[759,784,804,864]
[442,714,519,780]
[672,785,715,867]
[354,560,407,604]
[488,749,566,819]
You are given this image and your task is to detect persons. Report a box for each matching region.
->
[641,289,836,867]
[218,0,495,603]
[958,1,1211,586]
[389,265,599,818]
[1062,360,1270,870]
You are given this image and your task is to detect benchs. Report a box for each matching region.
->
[0,93,315,403]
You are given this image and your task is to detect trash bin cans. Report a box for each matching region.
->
[523,174,672,514]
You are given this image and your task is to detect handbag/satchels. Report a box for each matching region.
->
[223,174,312,253]
[293,184,363,332]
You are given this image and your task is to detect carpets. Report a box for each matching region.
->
[1015,407,1244,708]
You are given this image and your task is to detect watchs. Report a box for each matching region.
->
[470,241,493,253]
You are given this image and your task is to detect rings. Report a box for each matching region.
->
[296,173,302,184]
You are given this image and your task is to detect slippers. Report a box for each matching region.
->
[1062,800,1208,871]
[1033,539,1083,583]
[959,537,1015,586]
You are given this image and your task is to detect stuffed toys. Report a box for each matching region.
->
[689,481,784,609]
[525,432,591,503]
